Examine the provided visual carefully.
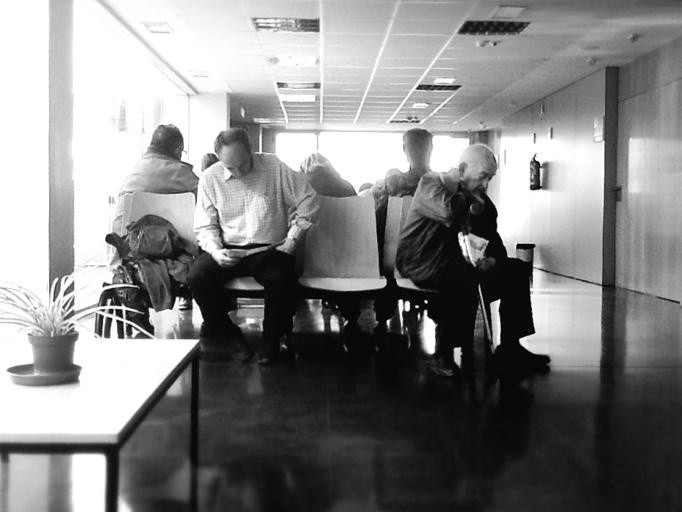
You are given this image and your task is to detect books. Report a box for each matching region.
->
[226,240,286,258]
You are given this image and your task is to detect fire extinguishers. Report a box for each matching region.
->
[529,154,541,190]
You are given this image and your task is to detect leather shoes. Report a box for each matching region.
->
[431,350,460,376]
[228,334,252,365]
[256,340,281,363]
[493,342,551,366]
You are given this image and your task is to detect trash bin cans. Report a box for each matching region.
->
[515,242,536,265]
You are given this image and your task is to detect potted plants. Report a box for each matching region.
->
[0,269,158,387]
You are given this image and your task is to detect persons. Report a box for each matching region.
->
[201,153,218,172]
[184,127,320,369]
[299,152,362,337]
[359,182,374,193]
[107,124,200,310]
[386,167,401,179]
[392,141,552,380]
[370,128,436,331]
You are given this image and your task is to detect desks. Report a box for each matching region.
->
[0,338,202,512]
